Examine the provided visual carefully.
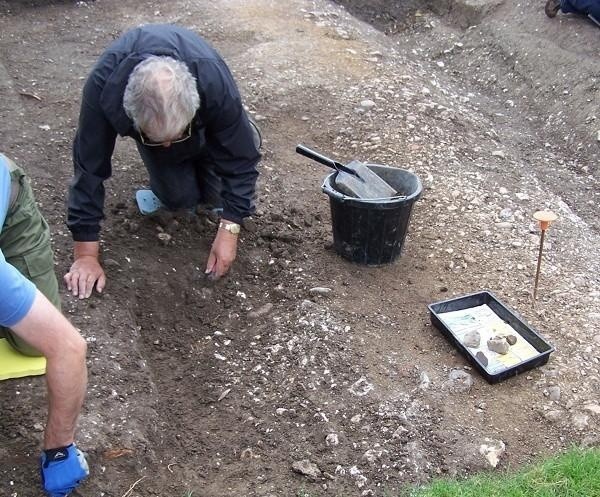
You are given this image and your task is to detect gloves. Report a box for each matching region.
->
[39,442,91,496]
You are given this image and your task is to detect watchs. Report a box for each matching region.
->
[217,220,242,234]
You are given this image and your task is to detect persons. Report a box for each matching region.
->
[60,22,263,301]
[0,151,91,496]
[544,0,600,26]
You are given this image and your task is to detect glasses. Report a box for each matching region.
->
[139,121,192,147]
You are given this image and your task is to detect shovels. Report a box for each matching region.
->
[296,144,398,201]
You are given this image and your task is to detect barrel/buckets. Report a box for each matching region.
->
[321,165,422,265]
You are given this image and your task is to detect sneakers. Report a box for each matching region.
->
[248,116,264,150]
